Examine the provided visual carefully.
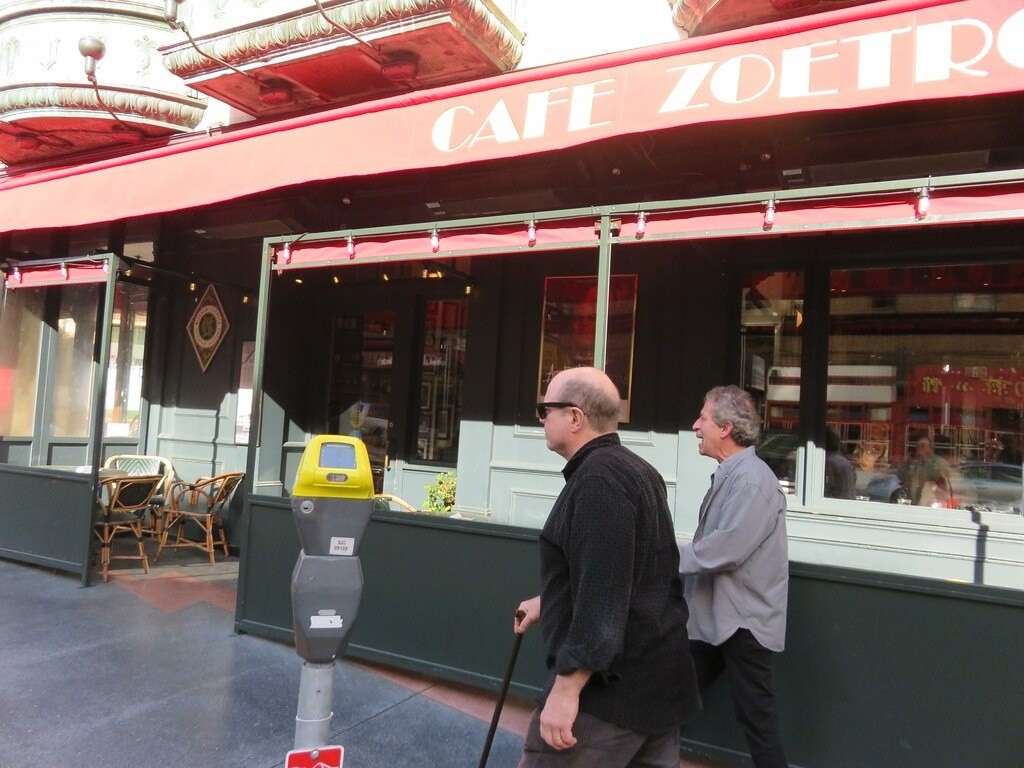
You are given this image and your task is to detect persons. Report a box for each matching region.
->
[675,385,788,768]
[825,429,962,509]
[513,367,702,768]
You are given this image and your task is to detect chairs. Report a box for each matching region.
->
[153,472,245,567]
[92,475,163,582]
[102,455,174,543]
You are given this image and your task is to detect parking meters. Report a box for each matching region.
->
[285,434,377,768]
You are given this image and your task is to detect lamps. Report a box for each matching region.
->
[425,200,446,210]
[525,220,538,241]
[637,212,650,232]
[77,36,127,131]
[282,234,292,258]
[914,187,934,213]
[344,237,355,254]
[760,152,776,161]
[163,0,272,89]
[337,195,352,209]
[428,229,440,248]
[762,200,780,224]
[192,224,208,234]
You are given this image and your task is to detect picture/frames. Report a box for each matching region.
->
[535,274,638,423]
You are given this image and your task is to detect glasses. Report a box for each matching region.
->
[536,402,585,419]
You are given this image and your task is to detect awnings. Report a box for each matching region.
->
[0,1,1023,234]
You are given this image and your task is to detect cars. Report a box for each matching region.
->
[752,432,857,494]
[867,461,1022,505]
